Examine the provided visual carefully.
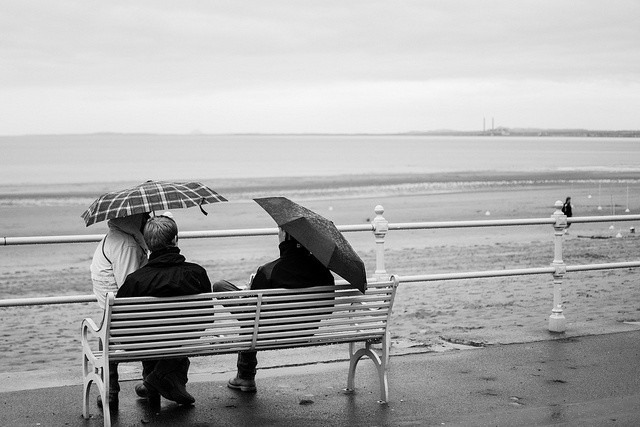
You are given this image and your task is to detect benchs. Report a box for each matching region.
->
[80,274,399,426]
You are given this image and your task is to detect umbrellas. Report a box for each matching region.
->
[80,179,228,228]
[252,197,368,294]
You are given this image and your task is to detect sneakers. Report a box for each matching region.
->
[97,395,118,414]
[143,366,195,404]
[228,375,256,392]
[135,385,160,397]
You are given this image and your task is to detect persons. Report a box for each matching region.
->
[96,214,215,410]
[561,196,573,234]
[90,211,197,404]
[212,224,336,393]
